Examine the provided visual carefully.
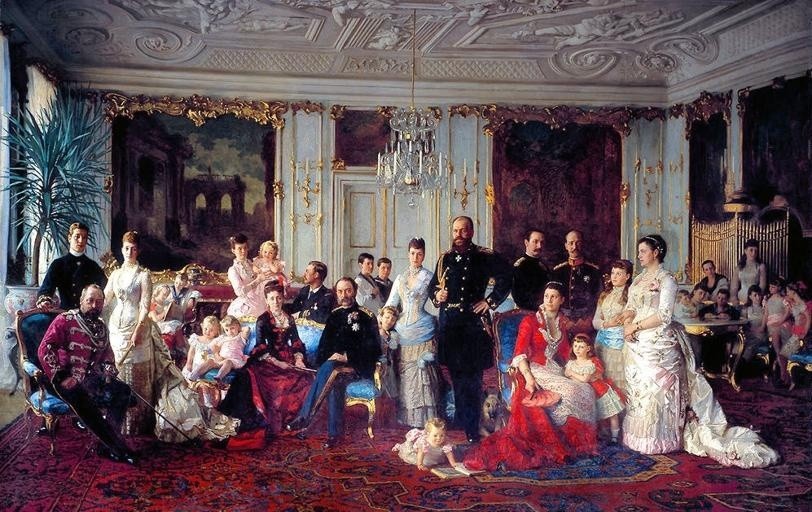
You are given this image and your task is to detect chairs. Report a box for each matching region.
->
[17,296,136,460]
[481,309,541,426]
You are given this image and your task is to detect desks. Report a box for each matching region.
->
[189,283,303,305]
[675,317,764,393]
[5,330,25,396]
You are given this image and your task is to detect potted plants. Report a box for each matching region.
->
[1,78,118,333]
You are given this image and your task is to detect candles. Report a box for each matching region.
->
[453,173,456,190]
[375,141,449,181]
[305,158,309,174]
[463,159,466,177]
[718,148,735,175]
[654,168,658,186]
[642,157,646,177]
[473,160,477,178]
[315,168,319,184]
[296,162,300,181]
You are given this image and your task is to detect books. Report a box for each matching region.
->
[431,464,487,479]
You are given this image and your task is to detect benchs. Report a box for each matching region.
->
[198,315,385,441]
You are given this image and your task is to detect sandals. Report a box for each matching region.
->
[608,436,620,448]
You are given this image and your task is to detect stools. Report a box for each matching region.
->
[785,350,812,390]
[751,343,773,376]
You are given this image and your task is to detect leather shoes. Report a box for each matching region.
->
[71,416,87,433]
[114,446,140,465]
[36,421,59,435]
[286,417,309,432]
[324,432,345,448]
[464,427,481,443]
[97,447,121,463]
